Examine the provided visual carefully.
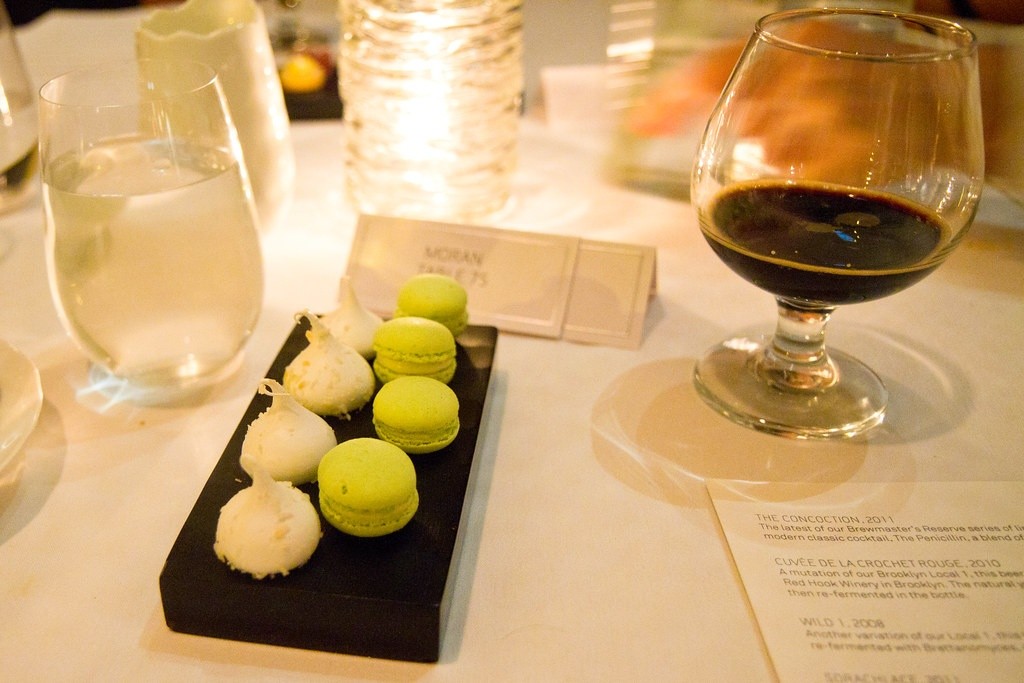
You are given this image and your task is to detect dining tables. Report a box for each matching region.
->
[0,1,1024,682]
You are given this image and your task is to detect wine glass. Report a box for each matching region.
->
[684,7,989,440]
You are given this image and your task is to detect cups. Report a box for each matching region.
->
[0,0,42,204]
[336,0,528,228]
[135,2,288,224]
[36,62,264,391]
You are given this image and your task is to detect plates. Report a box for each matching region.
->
[157,310,506,663]
[0,337,47,471]
